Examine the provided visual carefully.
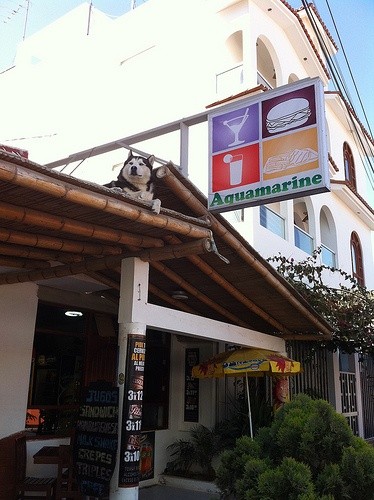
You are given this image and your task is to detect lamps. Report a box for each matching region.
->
[172,291,188,300]
[64,308,83,316]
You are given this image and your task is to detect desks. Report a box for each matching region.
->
[33,445,82,500]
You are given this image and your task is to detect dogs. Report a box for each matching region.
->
[102,148,162,215]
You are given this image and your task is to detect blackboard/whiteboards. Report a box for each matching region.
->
[69,387,121,495]
[184,347,200,423]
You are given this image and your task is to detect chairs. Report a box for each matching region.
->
[56,444,87,500]
[15,435,57,500]
